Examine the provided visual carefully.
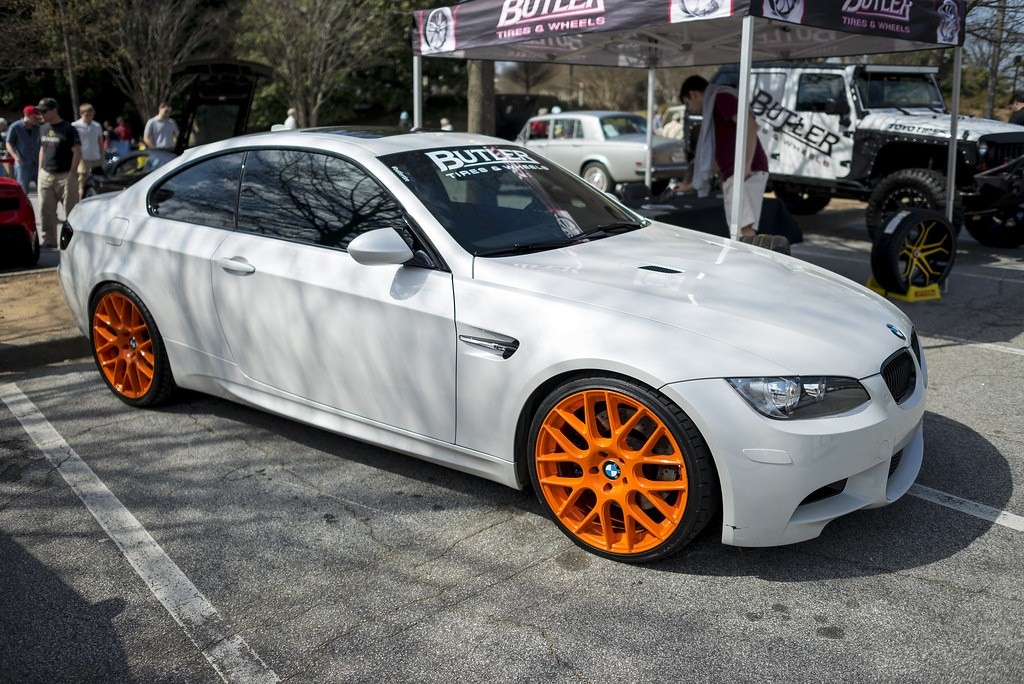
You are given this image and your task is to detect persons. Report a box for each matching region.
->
[5,106,41,196]
[143,102,180,150]
[530,104,562,135]
[71,104,104,198]
[33,98,82,249]
[672,74,769,235]
[439,116,454,131]
[398,111,412,128]
[1008,94,1024,126]
[102,116,136,160]
[0,117,15,179]
[284,108,298,129]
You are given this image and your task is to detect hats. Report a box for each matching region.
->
[33,98,57,111]
[24,106,40,116]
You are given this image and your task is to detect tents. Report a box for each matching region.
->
[412,0,968,243]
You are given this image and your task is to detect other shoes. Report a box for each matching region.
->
[40,243,58,249]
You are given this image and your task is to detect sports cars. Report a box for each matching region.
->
[58,123,930,567]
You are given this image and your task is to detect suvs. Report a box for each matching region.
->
[680,58,1024,258]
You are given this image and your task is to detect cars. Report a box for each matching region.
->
[0,154,40,272]
[80,59,274,200]
[512,110,690,197]
